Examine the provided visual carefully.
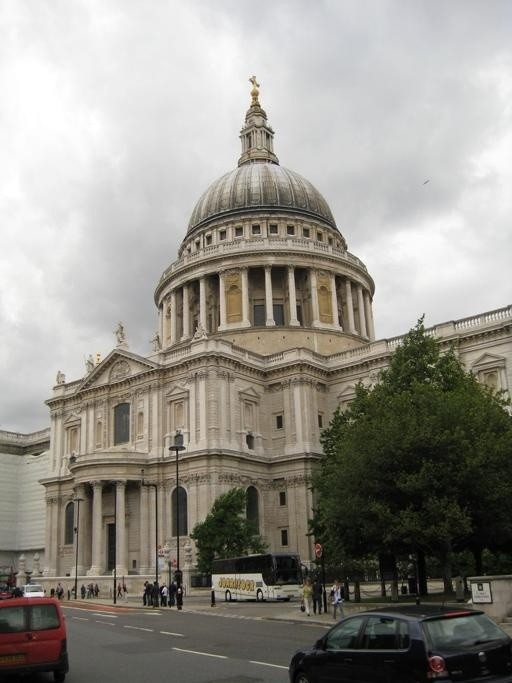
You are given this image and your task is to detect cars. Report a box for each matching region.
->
[288,604,512,683]
[0,584,69,683]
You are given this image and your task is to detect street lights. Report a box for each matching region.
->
[110,479,128,604]
[74,496,84,599]
[169,445,186,588]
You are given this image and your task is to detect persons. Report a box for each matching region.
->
[50,581,183,610]
[153,332,159,352]
[85,354,94,371]
[116,323,125,343]
[312,580,322,615]
[301,578,314,616]
[331,579,345,619]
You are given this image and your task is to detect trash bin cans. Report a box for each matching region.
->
[407,577,417,594]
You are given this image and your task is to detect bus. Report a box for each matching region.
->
[211,552,308,602]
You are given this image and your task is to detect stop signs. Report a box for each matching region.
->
[315,543,323,558]
